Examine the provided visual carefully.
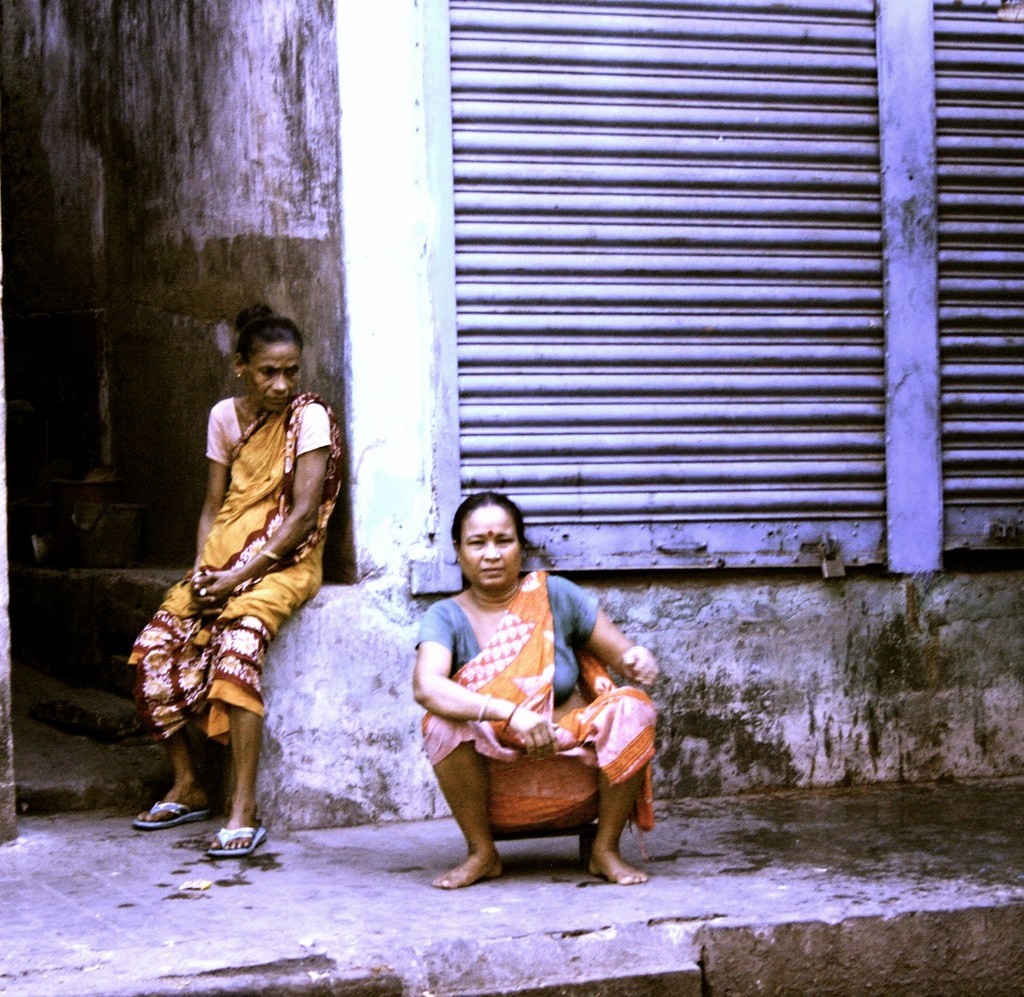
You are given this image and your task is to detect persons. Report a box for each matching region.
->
[413,492,661,889]
[133,305,343,858]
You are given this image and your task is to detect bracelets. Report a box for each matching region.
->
[503,706,519,730]
[478,694,492,722]
[260,551,281,561]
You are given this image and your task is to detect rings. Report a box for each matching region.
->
[199,589,206,596]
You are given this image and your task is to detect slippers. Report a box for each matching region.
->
[207,826,267,855]
[131,801,212,827]
